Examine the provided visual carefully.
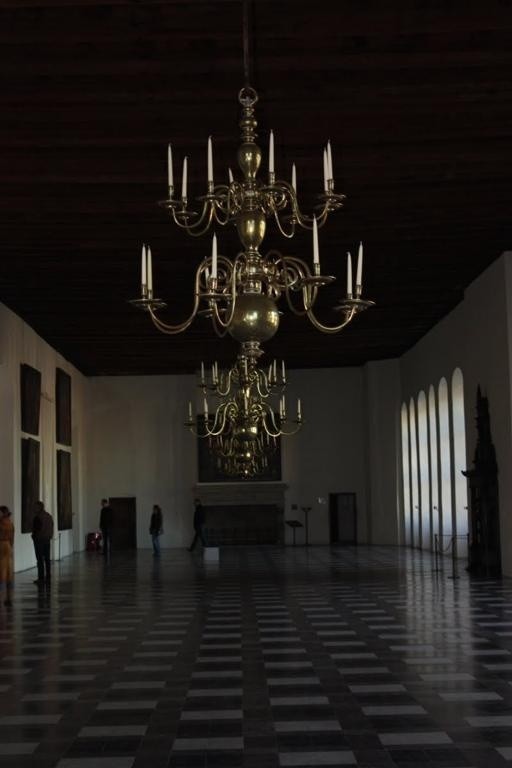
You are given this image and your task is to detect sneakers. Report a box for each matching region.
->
[33,576,51,585]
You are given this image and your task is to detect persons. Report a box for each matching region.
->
[30,501,53,584]
[186,498,207,552]
[1,505,15,607]
[148,504,165,553]
[98,499,115,554]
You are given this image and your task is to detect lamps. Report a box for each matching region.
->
[128,0,376,480]
